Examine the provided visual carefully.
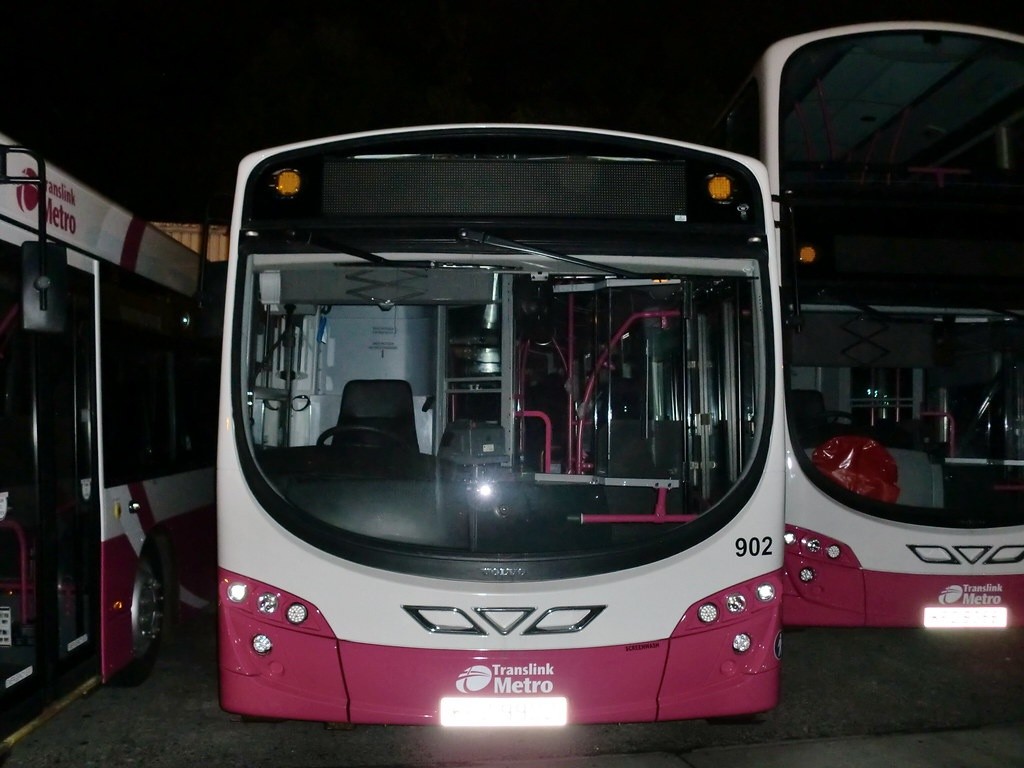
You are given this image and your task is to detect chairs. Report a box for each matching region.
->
[453,371,684,475]
[791,388,829,439]
[331,380,423,453]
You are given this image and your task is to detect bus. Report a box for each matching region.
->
[0,134,228,747]
[696,16,1024,639]
[210,118,807,735]
